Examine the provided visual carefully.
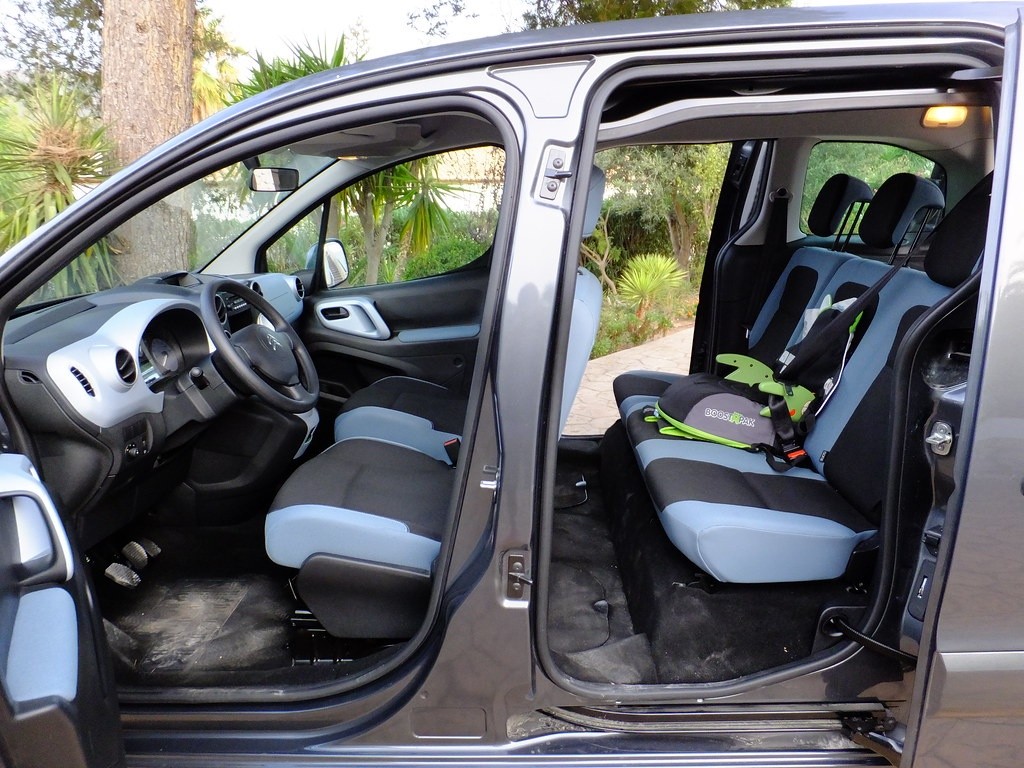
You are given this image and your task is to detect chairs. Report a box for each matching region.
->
[332,375,468,466]
[263,435,457,576]
[611,168,996,586]
